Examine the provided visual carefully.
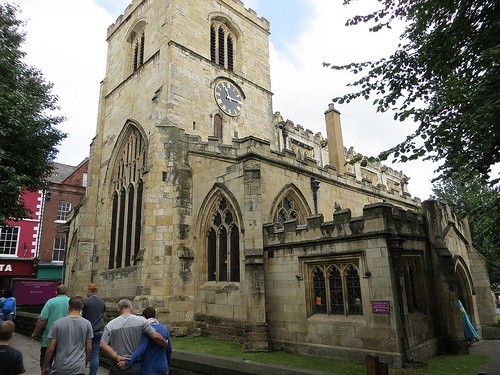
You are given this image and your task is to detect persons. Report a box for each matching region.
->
[100,298,168,375]
[41,296,94,375]
[0,291,16,335]
[32,285,71,370]
[0,320,26,375]
[116,307,172,375]
[82,284,106,375]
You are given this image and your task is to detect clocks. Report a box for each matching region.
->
[214,80,244,118]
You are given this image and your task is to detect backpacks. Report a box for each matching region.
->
[3,298,15,313]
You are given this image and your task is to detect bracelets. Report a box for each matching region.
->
[112,354,118,361]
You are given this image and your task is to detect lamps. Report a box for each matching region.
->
[362,271,372,279]
[295,275,303,281]
